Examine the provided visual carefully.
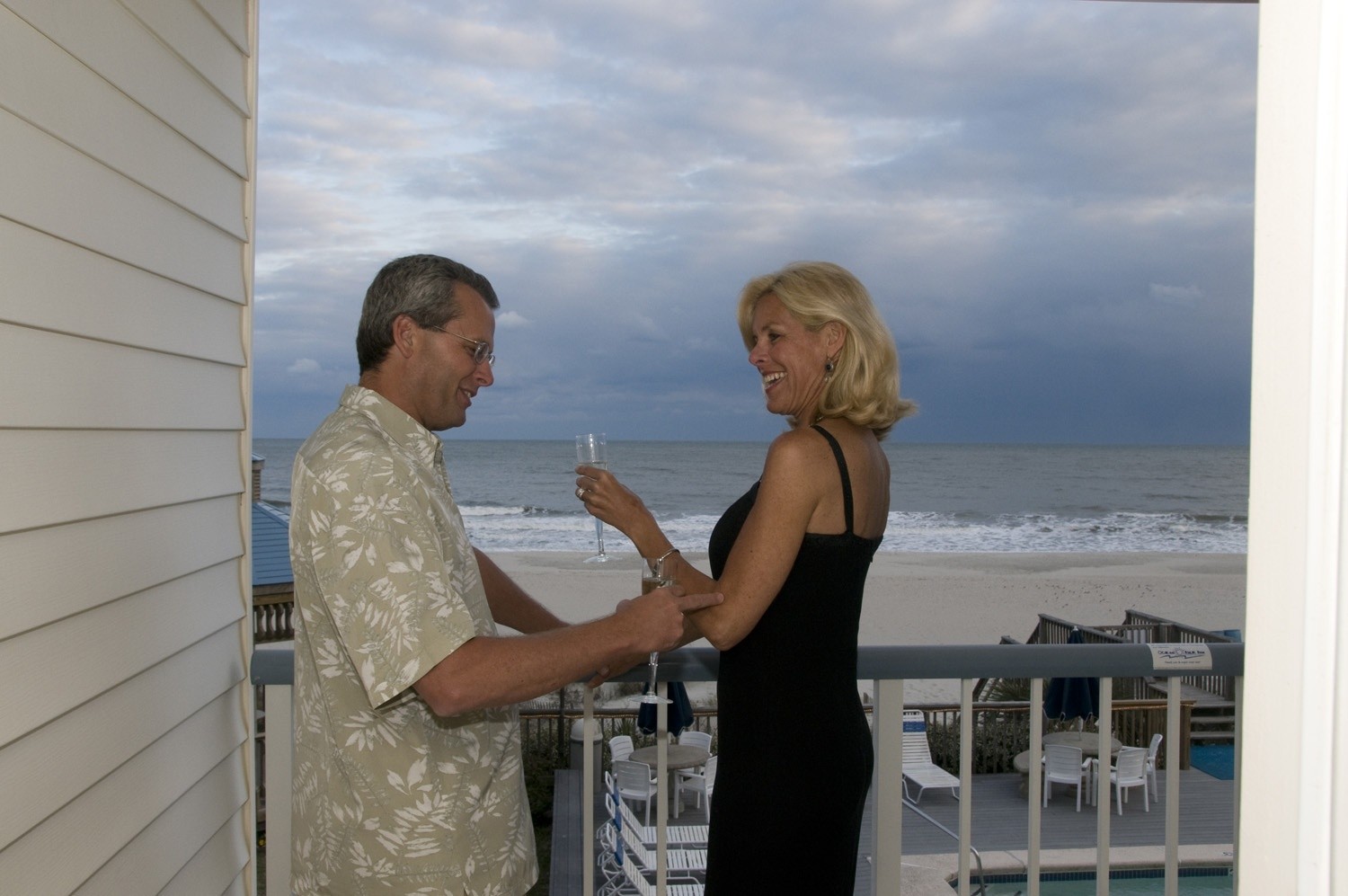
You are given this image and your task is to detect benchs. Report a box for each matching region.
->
[596,771,709,896]
[903,710,960,805]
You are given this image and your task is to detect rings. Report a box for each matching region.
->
[577,488,586,498]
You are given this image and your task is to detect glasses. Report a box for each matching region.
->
[424,321,496,369]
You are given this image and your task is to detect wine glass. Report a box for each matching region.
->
[575,432,623,563]
[627,557,676,705]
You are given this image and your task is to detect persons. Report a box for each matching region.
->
[290,253,723,896]
[578,261,916,896]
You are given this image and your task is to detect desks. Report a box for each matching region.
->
[629,745,710,813]
[1042,732,1122,798]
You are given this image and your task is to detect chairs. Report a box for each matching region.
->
[609,736,633,779]
[679,732,712,752]
[615,761,657,828]
[1041,743,1090,813]
[1122,734,1164,803]
[1092,748,1151,816]
[673,756,719,823]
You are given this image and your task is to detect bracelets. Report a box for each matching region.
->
[654,548,679,574]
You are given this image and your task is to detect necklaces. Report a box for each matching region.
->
[807,415,826,425]
